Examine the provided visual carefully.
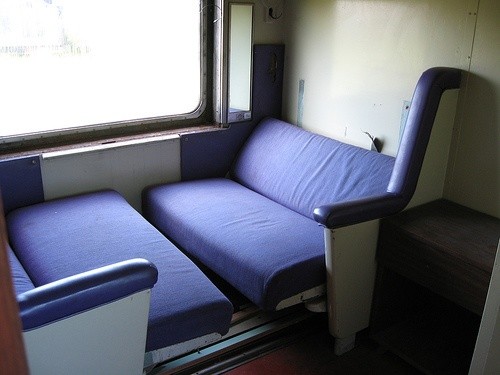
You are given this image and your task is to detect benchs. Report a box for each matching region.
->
[141,115,397,319]
[3,188,236,366]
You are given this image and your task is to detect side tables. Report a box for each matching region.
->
[354,196,500,375]
